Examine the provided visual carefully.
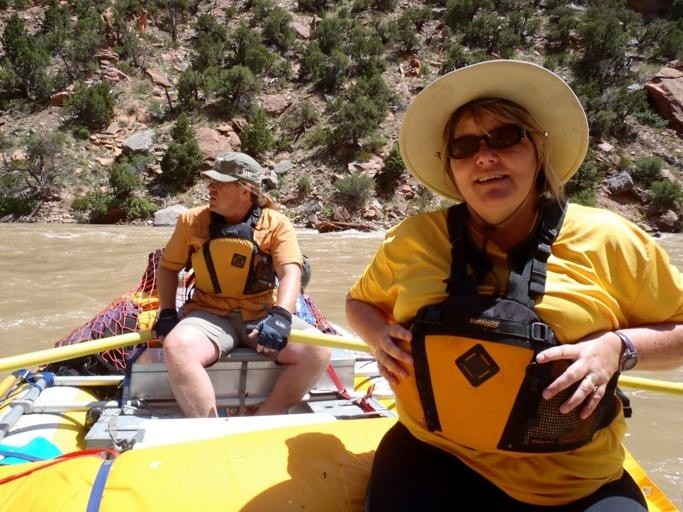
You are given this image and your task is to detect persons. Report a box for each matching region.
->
[343,59,683,511]
[150,152,330,418]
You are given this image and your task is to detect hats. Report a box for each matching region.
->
[199,151,263,187]
[399,60,590,202]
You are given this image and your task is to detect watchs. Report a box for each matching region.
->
[614,329,637,373]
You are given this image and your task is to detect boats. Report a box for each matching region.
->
[1,317,683,512]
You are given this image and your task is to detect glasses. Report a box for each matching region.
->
[445,124,525,160]
